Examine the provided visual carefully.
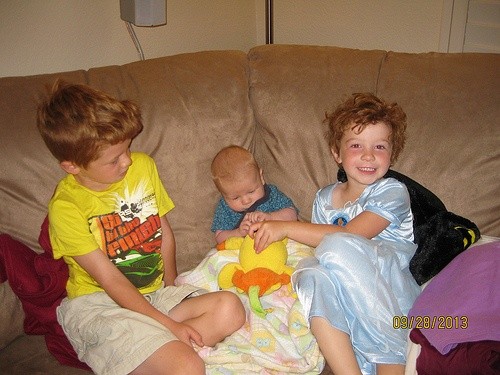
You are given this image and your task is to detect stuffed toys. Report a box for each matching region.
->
[215,232,297,319]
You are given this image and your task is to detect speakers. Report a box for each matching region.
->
[119,0,167,27]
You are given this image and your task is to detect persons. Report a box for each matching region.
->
[209,145,299,243]
[35,78,246,375]
[248,93,420,375]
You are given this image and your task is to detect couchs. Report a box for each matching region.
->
[0,44,500,375]
